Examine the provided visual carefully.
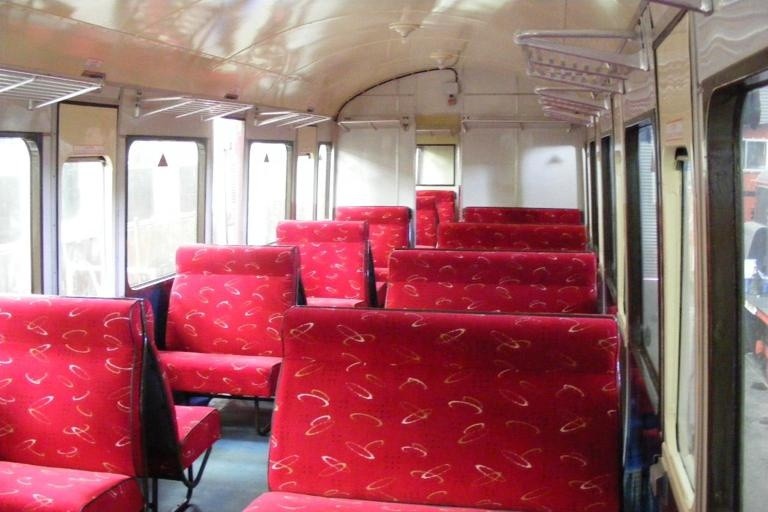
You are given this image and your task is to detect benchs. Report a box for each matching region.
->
[0,188,629,512]
